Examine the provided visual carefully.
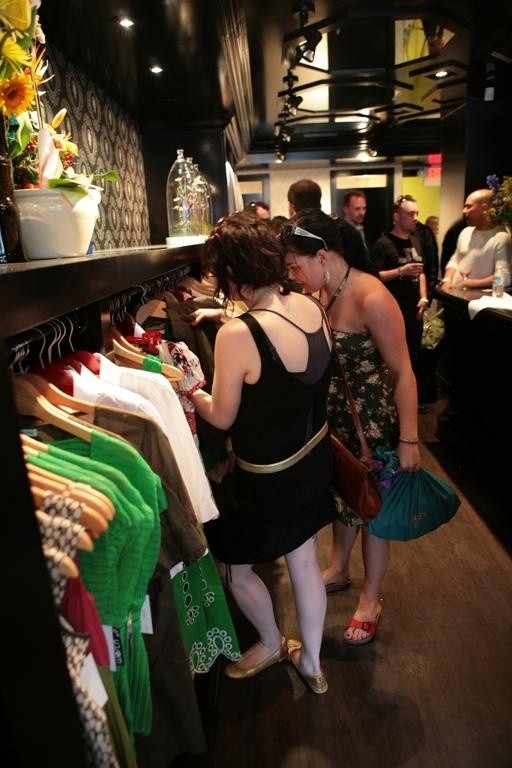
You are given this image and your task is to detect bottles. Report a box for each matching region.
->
[491,266,504,298]
[165,147,213,248]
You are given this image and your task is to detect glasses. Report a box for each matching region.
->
[279,223,334,251]
[396,193,417,205]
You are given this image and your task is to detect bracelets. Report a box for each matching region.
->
[398,437,419,443]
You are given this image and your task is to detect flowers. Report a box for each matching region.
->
[10,106,120,210]
[481,172,511,235]
[0,0,59,122]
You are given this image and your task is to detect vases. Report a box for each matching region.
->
[12,183,105,261]
[0,104,28,265]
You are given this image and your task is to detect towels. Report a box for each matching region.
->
[467,290,511,321]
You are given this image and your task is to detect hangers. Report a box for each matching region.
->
[7,263,220,580]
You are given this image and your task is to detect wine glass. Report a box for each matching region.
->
[460,268,472,291]
[409,256,424,282]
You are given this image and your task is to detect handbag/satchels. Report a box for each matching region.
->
[366,452,463,543]
[324,427,381,521]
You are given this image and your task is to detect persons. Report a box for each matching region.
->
[188,211,341,694]
[278,212,420,645]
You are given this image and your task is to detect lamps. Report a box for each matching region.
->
[272,0,324,164]
